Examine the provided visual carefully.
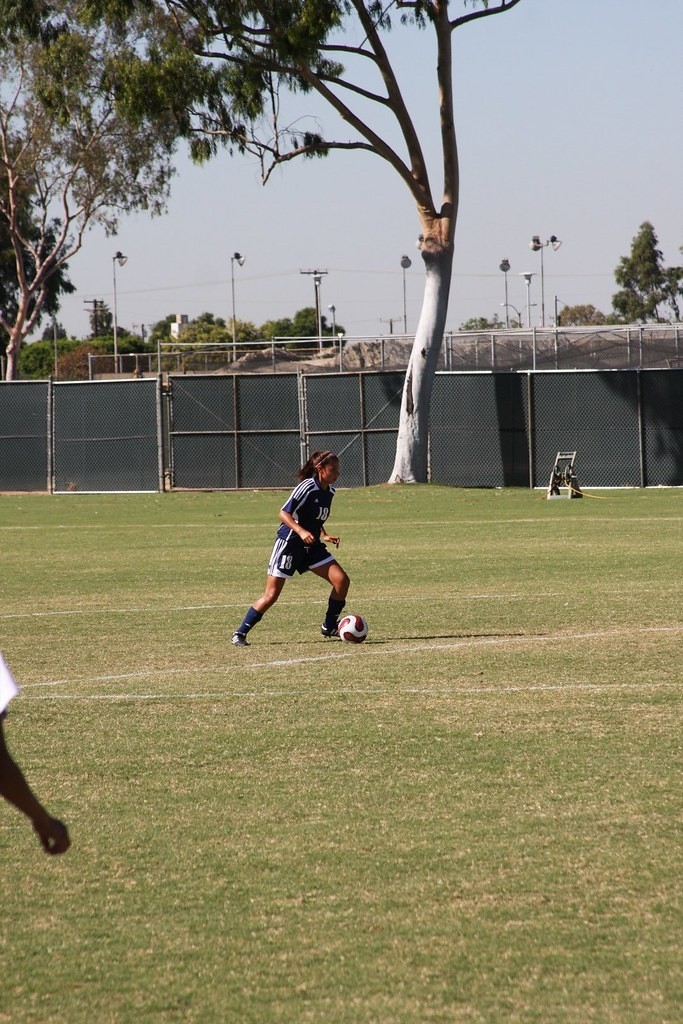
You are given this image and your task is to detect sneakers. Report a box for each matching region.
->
[231,631,252,647]
[321,620,341,638]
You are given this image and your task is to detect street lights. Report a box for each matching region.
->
[129,353,139,369]
[113,250,128,373]
[313,275,323,351]
[48,314,59,381]
[230,252,246,362]
[529,235,563,329]
[499,259,512,329]
[519,272,536,329]
[327,304,337,348]
[501,301,538,358]
[132,322,154,341]
[400,255,413,335]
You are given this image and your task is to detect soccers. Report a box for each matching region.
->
[338,615,369,643]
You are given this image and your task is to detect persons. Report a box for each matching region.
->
[0,656,71,856]
[231,450,350,647]
